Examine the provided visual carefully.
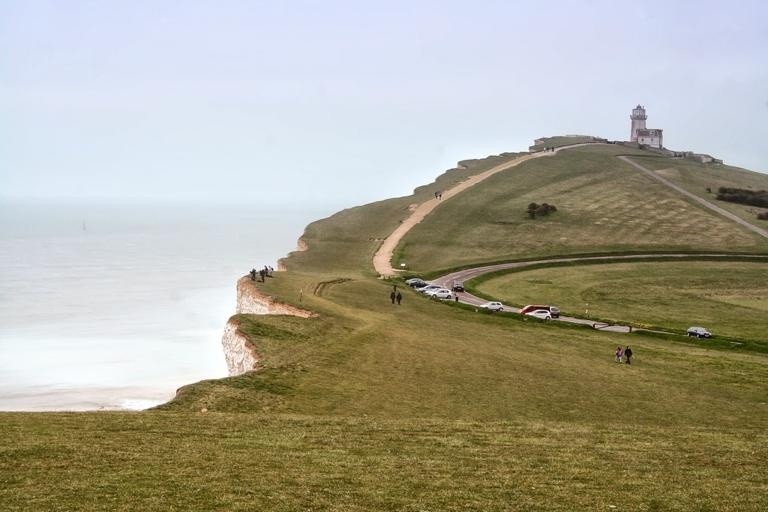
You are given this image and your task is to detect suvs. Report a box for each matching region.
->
[481,302,504,312]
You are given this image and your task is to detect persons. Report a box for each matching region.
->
[390,291,395,304]
[454,292,459,302]
[625,346,632,364]
[543,145,555,152]
[615,346,623,363]
[249,265,274,283]
[435,191,442,200]
[396,292,402,305]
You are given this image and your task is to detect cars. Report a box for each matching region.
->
[517,305,559,320]
[406,276,464,300]
[685,327,710,338]
